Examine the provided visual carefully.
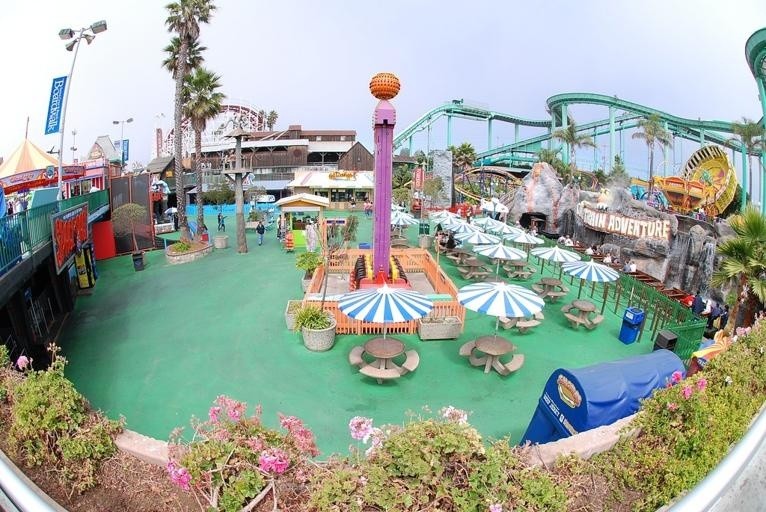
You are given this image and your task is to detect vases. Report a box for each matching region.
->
[418,316,462,341]
[285,299,303,330]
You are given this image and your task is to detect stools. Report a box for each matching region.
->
[349,345,365,365]
[358,364,400,385]
[433,236,605,376]
[402,350,420,372]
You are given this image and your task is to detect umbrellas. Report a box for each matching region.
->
[432,209,526,233]
[482,200,510,214]
[562,256,620,299]
[454,230,503,246]
[391,202,419,240]
[472,242,529,277]
[336,281,433,343]
[503,228,545,251]
[530,244,582,279]
[164,206,179,223]
[457,281,547,341]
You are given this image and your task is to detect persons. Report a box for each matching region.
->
[275,215,282,240]
[216,214,228,233]
[349,197,356,213]
[370,202,373,214]
[363,200,371,216]
[559,235,636,273]
[255,221,266,246]
[172,212,180,231]
[433,224,457,256]
[682,289,730,332]
[216,213,223,228]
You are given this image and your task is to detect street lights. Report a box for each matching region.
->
[113,117,133,150]
[58,20,108,200]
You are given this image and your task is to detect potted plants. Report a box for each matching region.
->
[293,303,337,352]
[294,250,328,293]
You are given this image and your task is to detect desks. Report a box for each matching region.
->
[359,337,409,385]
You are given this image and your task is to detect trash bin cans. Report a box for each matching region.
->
[652,329,677,351]
[213,236,229,248]
[132,251,144,271]
[618,306,645,345]
